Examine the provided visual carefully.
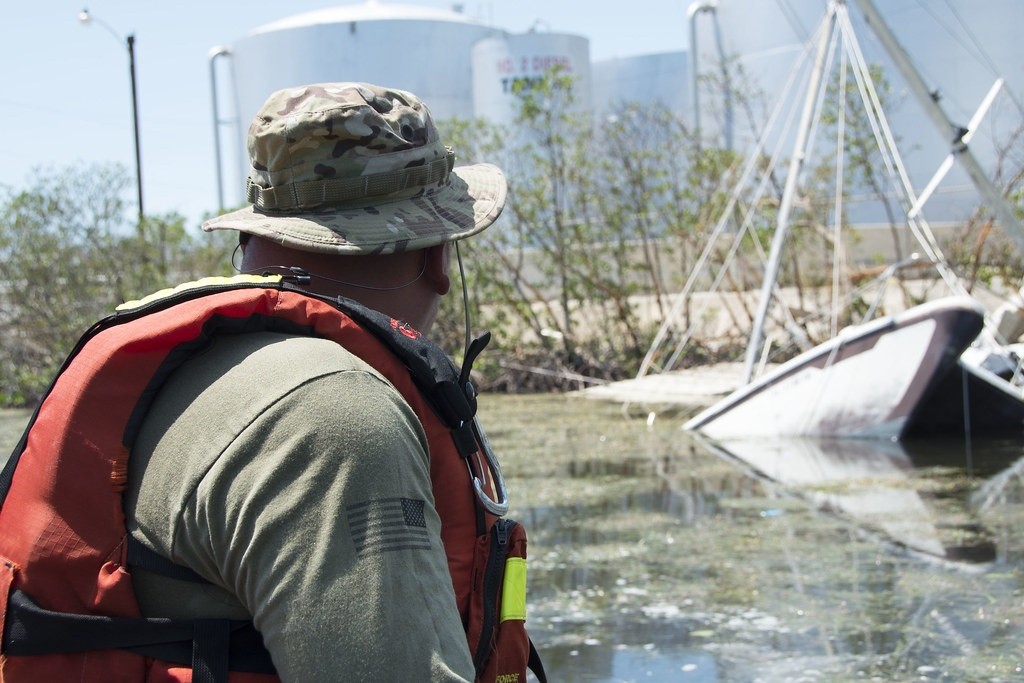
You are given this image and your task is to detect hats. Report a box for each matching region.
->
[201,82,507,255]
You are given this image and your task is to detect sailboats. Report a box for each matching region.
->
[565,0,1024,452]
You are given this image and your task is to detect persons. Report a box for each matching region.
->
[0,84,548,683]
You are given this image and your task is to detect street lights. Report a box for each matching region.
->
[77,9,144,234]
